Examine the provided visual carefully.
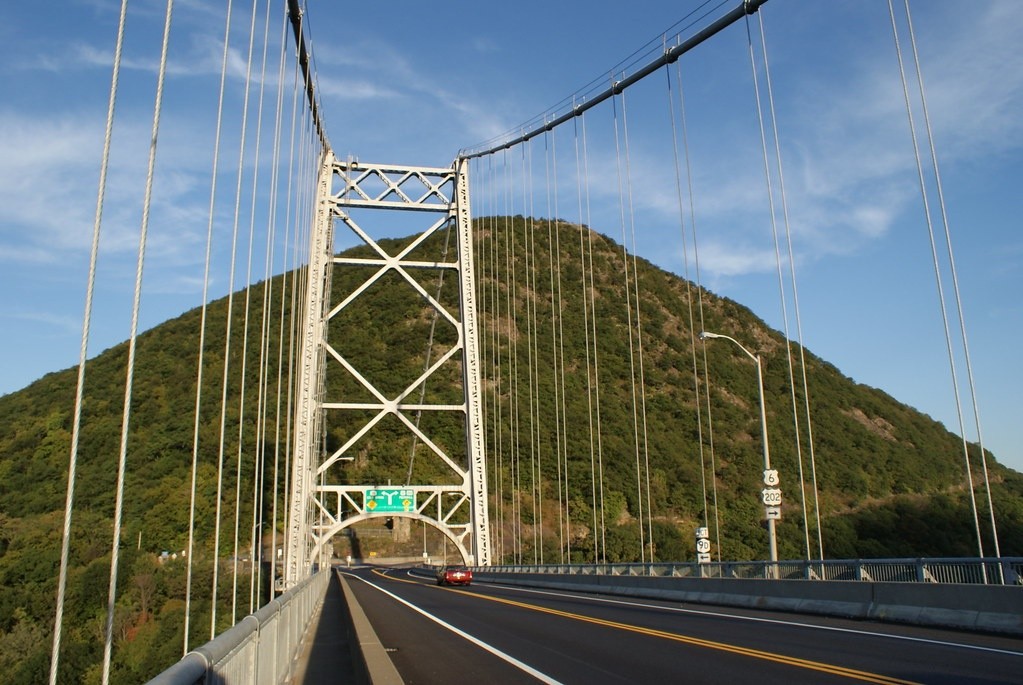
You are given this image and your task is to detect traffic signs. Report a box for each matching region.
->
[362,488,416,514]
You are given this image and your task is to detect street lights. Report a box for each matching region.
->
[700,331,782,581]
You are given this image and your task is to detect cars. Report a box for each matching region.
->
[437,564,473,586]
